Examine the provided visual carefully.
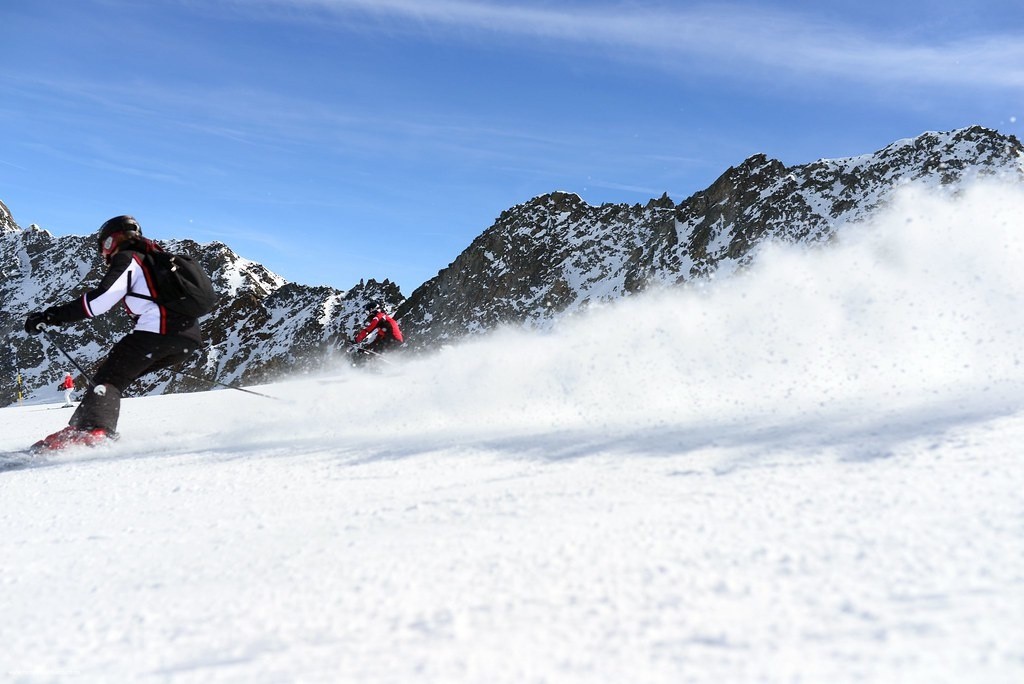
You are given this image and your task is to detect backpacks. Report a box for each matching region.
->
[113,249,216,318]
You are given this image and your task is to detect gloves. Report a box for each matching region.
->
[25,312,54,335]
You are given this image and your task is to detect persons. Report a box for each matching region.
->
[24,214,201,453]
[62,372,74,406]
[353,302,404,369]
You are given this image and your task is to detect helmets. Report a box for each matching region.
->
[65,372,70,377]
[98,215,142,264]
[365,302,382,317]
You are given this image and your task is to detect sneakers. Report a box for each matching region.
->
[43,425,72,444]
[51,427,107,450]
